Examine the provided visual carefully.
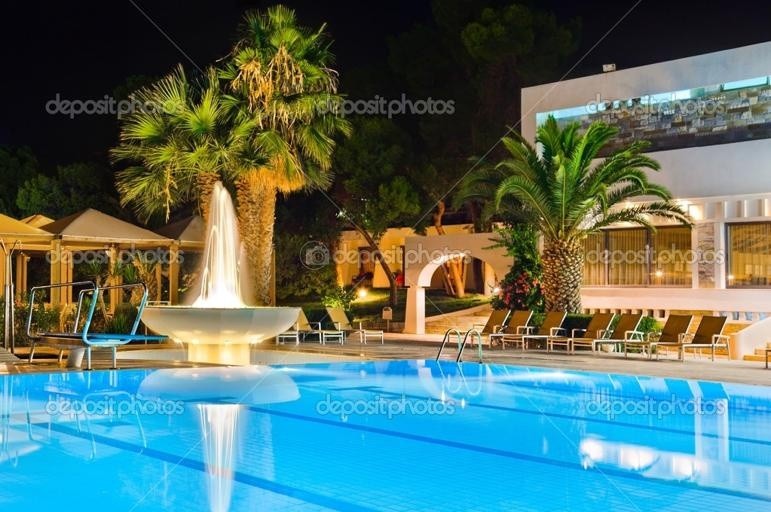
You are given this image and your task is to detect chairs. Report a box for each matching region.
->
[447,307,734,364]
[272,306,386,347]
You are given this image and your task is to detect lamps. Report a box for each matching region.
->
[758,198,771,217]
[737,199,749,218]
[714,199,729,220]
